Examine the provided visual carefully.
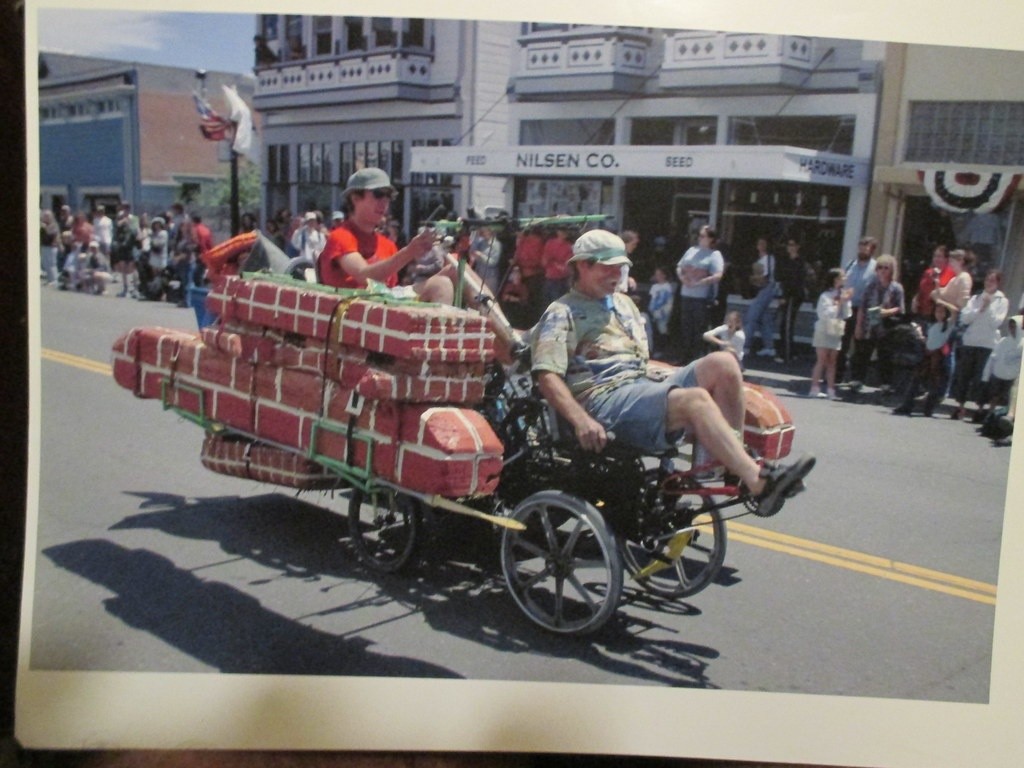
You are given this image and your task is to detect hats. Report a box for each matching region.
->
[565,228,636,268]
[340,168,400,199]
[304,212,316,222]
[332,210,344,220]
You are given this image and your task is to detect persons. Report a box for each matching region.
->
[849,256,905,403]
[615,230,639,292]
[950,270,1010,422]
[648,266,674,358]
[542,227,574,302]
[809,268,852,400]
[773,236,806,365]
[701,311,746,375]
[531,231,814,518]
[931,249,973,330]
[896,300,958,418]
[979,315,1022,428]
[744,237,781,358]
[42,203,401,300]
[514,226,548,329]
[911,246,948,324]
[676,225,724,365]
[410,227,443,283]
[318,168,529,368]
[468,226,502,303]
[825,236,876,383]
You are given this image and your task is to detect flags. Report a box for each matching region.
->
[192,90,234,140]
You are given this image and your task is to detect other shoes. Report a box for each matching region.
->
[849,379,863,389]
[952,409,965,420]
[924,401,933,416]
[881,384,890,396]
[895,405,915,414]
[757,348,777,356]
[829,390,843,400]
[973,411,986,423]
[808,388,826,397]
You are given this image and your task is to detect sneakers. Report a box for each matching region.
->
[726,449,807,494]
[757,454,818,515]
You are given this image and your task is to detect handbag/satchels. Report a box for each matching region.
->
[682,265,710,286]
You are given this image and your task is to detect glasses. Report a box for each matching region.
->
[879,266,889,270]
[364,189,393,200]
